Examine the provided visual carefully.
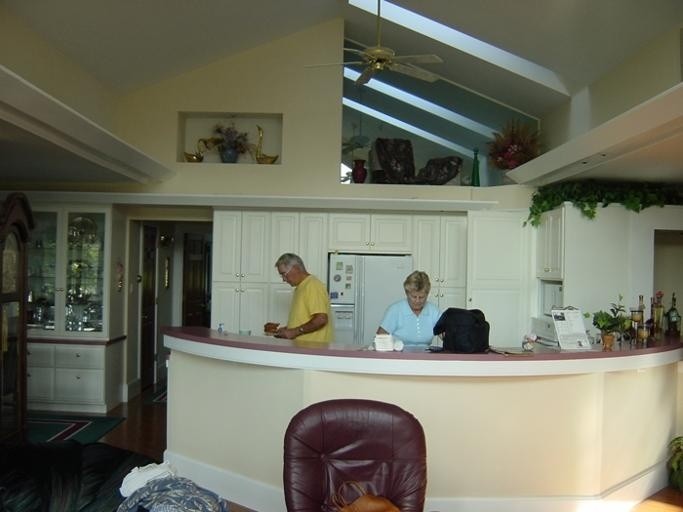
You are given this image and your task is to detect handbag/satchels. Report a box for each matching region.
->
[434,305,491,353]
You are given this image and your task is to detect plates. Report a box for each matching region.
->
[70,216,97,248]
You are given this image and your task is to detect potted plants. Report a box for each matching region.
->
[585,295,629,348]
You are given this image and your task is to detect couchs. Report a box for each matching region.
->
[284,399,426,511]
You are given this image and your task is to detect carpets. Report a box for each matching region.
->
[0,408,125,445]
[144,387,167,405]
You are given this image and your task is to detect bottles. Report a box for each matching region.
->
[83,310,89,328]
[666,292,681,345]
[651,291,665,342]
[638,296,644,309]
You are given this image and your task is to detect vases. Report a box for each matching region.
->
[220,148,238,163]
[351,159,367,183]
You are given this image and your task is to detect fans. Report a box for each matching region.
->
[306,0,444,87]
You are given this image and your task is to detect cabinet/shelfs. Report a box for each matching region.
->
[270,212,327,283]
[269,283,297,338]
[328,213,413,254]
[532,208,628,348]
[212,209,270,283]
[413,215,467,347]
[27,203,126,417]
[210,282,269,338]
[467,210,532,347]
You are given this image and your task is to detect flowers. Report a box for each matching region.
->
[198,121,251,154]
[485,118,545,169]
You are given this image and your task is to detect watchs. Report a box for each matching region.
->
[299,326,305,334]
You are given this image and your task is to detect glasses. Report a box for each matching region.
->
[278,266,294,279]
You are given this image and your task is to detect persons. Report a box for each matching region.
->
[264,253,333,344]
[377,270,443,347]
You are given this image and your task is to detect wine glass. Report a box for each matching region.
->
[630,311,643,326]
[637,326,651,348]
[600,329,617,351]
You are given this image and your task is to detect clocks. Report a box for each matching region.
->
[0,192,35,445]
